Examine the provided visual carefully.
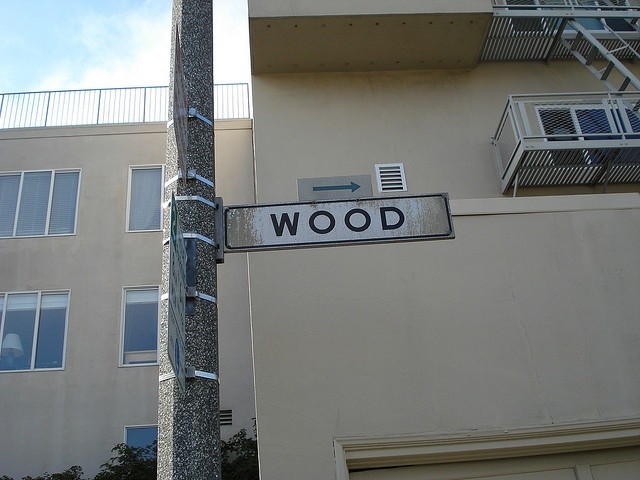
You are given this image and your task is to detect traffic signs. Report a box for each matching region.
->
[225,172,458,258]
